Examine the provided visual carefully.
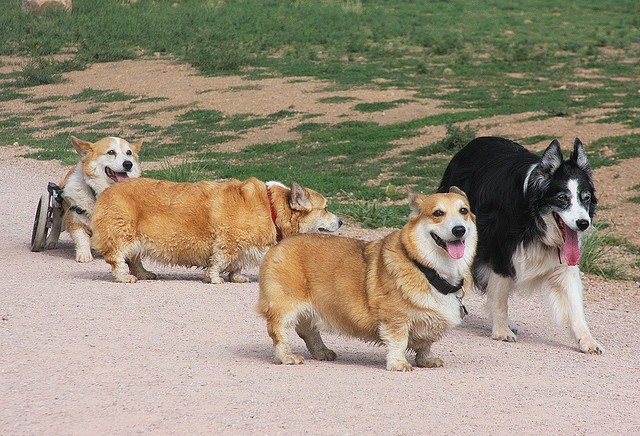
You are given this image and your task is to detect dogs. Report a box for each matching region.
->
[253,184,481,375]
[89,176,345,286]
[437,134,607,357]
[43,134,146,265]
